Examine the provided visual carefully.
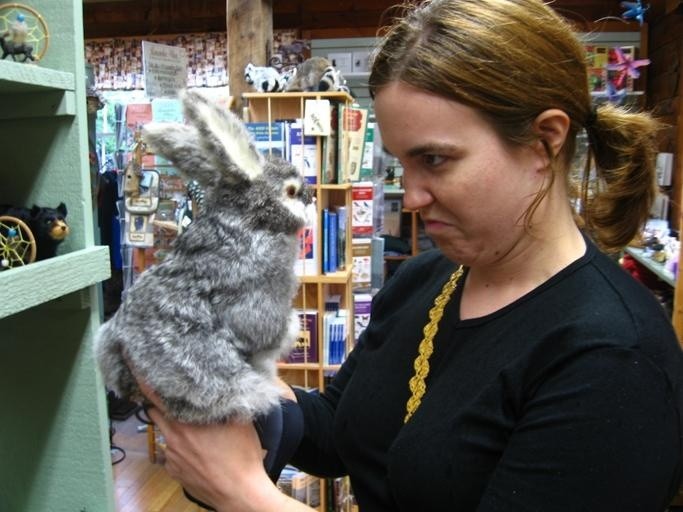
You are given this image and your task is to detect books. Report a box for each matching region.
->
[277,464,355,512]
[244,99,385,378]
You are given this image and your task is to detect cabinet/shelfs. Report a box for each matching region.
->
[1,1,114,512]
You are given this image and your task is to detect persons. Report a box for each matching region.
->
[121,0,683,512]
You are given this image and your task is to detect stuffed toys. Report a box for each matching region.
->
[88,87,315,429]
[1,203,70,271]
[241,55,349,93]
